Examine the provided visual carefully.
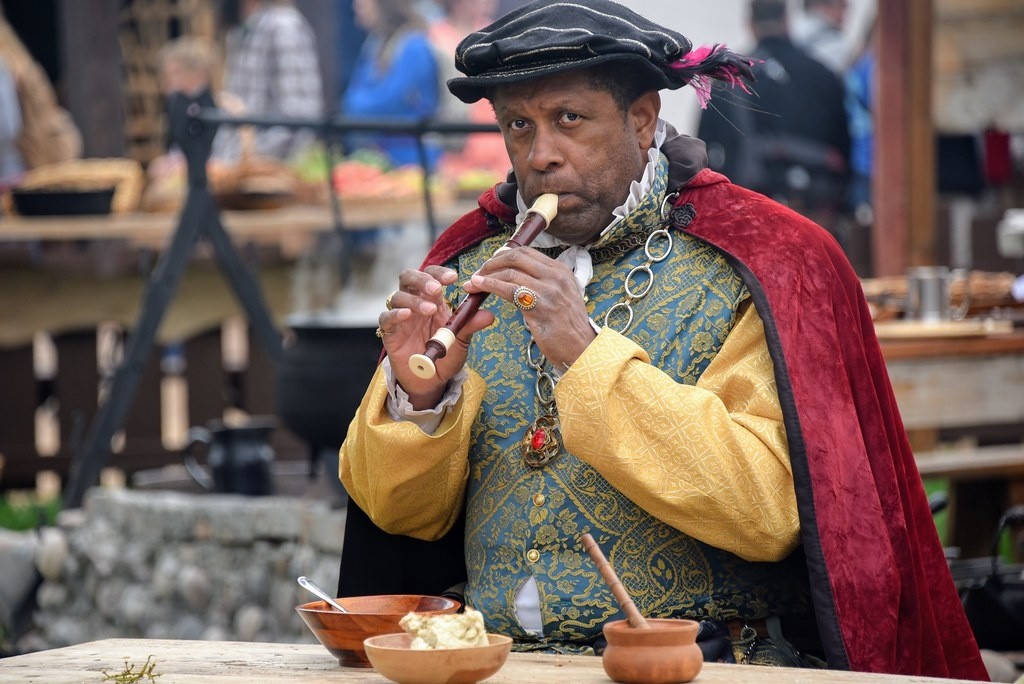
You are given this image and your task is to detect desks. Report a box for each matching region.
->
[868,324,1024,553]
[6,181,434,505]
[0,637,1013,684]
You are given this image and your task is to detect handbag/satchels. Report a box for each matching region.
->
[948,507,1024,650]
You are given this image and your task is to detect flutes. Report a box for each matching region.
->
[406,192,560,379]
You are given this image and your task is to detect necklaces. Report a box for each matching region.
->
[521,190,681,468]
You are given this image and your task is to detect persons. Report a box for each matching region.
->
[0,0,485,405]
[335,0,858,669]
[696,0,876,280]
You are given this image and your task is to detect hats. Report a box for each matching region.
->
[445,0,788,139]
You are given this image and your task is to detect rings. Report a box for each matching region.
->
[375,325,398,338]
[513,285,536,310]
[386,291,396,310]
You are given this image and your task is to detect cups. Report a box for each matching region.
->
[909,267,971,324]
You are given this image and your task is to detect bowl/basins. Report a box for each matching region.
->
[294,594,461,667]
[363,632,513,683]
